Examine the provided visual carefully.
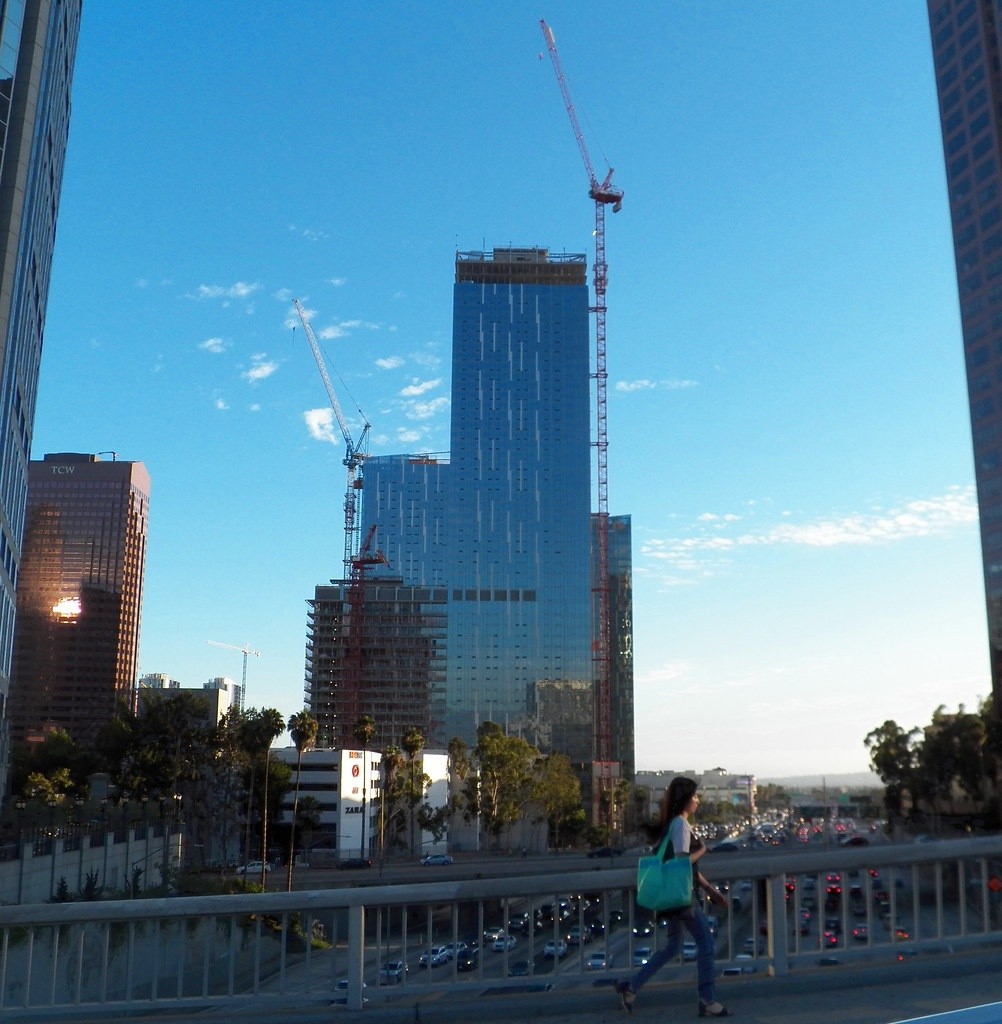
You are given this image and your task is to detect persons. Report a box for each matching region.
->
[614,776,735,1017]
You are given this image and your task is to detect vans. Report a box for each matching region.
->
[456,947,480,971]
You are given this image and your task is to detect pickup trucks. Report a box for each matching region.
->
[587,951,614,969]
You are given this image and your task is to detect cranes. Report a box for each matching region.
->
[290,297,396,750]
[206,637,260,715]
[535,13,626,828]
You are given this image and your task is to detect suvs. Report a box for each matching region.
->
[236,862,271,876]
[419,944,449,968]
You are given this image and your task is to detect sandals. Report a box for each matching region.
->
[697,999,734,1018]
[615,981,637,1016]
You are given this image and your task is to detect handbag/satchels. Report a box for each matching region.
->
[637,855,694,911]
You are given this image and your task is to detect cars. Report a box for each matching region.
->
[492,934,517,953]
[704,880,767,972]
[683,942,699,962]
[483,926,503,942]
[507,893,670,959]
[785,869,914,967]
[421,855,454,866]
[336,858,372,871]
[692,810,886,848]
[380,959,409,982]
[633,946,654,967]
[443,942,467,960]
[507,960,537,976]
